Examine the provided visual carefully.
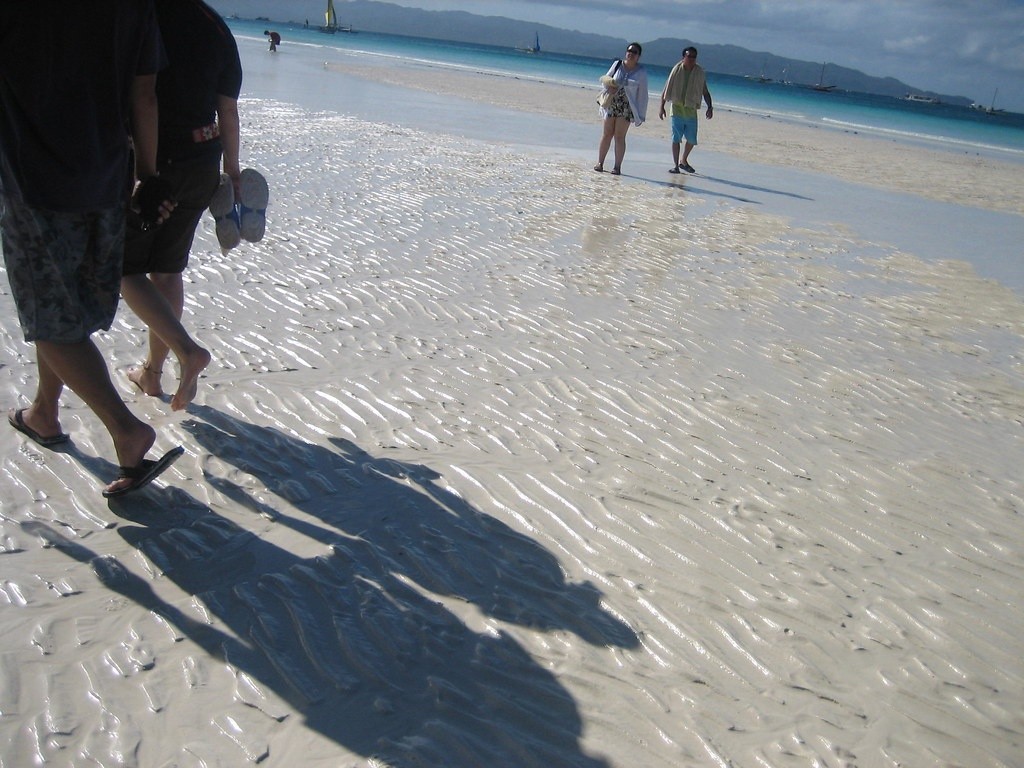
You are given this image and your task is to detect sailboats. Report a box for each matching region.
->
[513,30,541,55]
[317,0,339,33]
[984,87,1007,115]
[743,60,779,84]
[813,61,837,91]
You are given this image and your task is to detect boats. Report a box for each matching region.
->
[895,92,933,103]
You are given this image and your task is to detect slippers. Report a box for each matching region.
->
[101,446,183,497]
[670,169,681,173]
[679,163,695,173]
[8,409,70,446]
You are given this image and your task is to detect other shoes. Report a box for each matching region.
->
[594,163,603,171]
[210,172,241,249]
[611,168,620,174]
[238,168,269,242]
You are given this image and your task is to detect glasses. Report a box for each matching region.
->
[627,49,638,55]
[686,55,696,59]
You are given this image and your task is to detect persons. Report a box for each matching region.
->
[593,42,648,174]
[658,47,713,173]
[0,0,244,499]
[264,30,281,53]
